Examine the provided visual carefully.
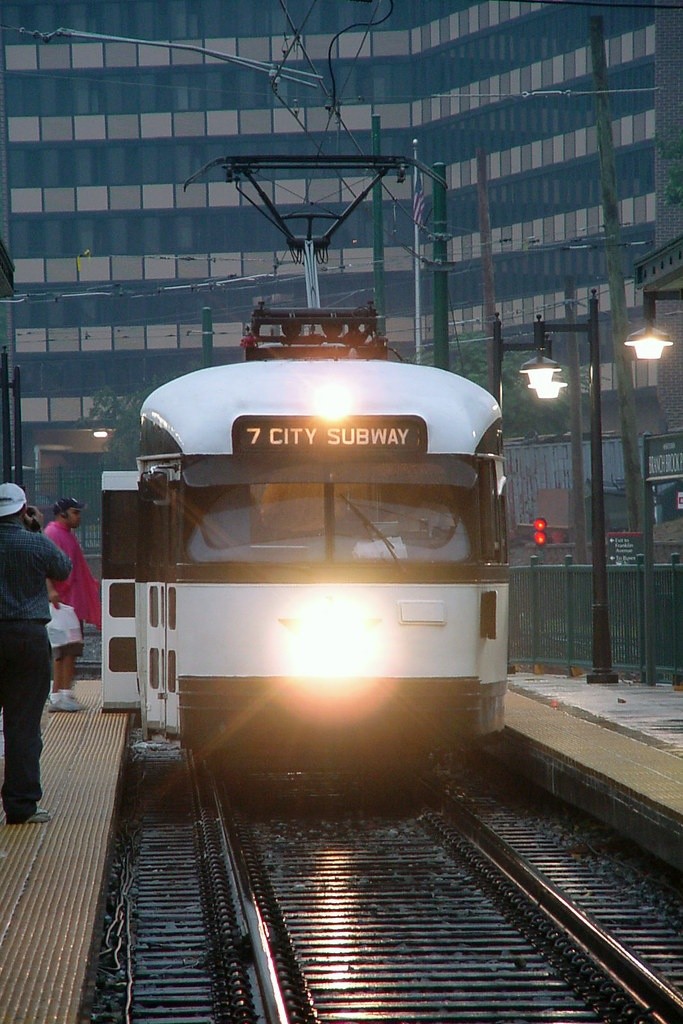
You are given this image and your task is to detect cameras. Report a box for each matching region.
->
[24,508,36,525]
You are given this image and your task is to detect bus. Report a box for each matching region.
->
[96,307,569,798]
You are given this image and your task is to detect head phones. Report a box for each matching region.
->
[55,502,69,518]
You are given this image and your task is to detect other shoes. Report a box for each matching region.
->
[25,808,52,823]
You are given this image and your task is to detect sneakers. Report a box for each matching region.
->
[48,690,83,713]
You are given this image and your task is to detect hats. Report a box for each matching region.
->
[53,496,84,516]
[0,483,27,517]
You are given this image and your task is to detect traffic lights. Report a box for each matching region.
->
[533,518,548,546]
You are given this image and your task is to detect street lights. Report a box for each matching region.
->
[534,314,618,683]
[492,339,566,409]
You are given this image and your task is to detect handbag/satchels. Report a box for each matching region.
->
[45,601,83,649]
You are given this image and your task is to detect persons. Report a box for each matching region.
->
[0,484,72,827]
[43,497,101,711]
